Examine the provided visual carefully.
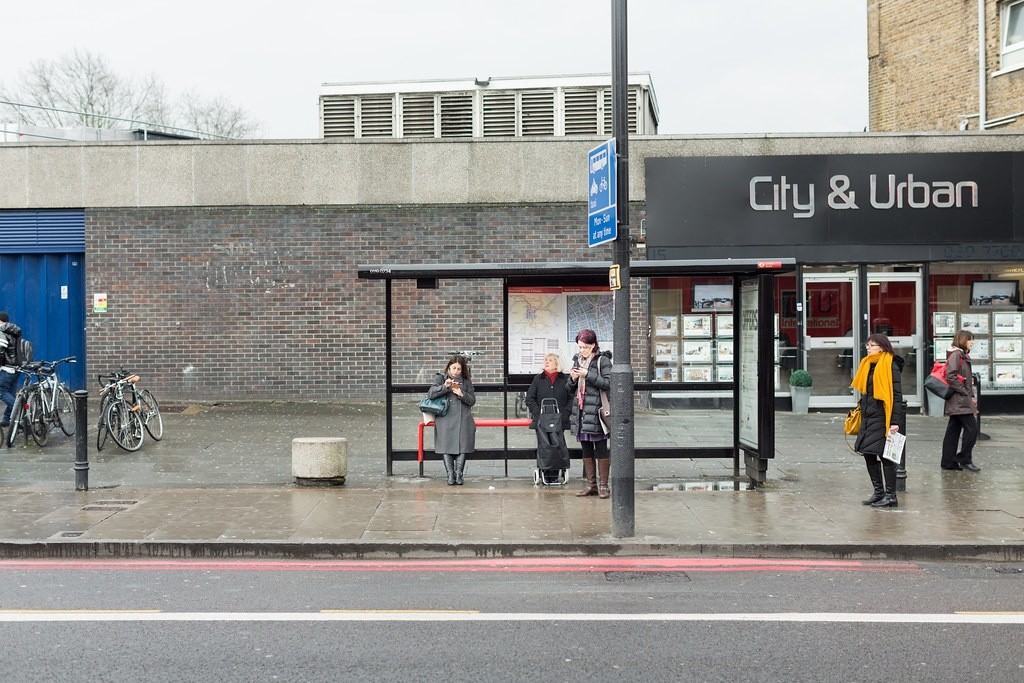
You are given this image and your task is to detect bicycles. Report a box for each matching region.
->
[6,356,78,447]
[97,365,163,451]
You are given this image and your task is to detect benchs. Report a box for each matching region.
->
[418,419,533,477]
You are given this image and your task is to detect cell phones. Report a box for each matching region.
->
[572,367,578,373]
[450,382,459,391]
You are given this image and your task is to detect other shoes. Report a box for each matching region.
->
[941,464,963,470]
[0,421,10,427]
[541,478,559,484]
[960,461,980,472]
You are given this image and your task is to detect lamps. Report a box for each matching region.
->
[475,77,491,86]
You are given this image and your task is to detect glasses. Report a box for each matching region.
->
[865,343,880,350]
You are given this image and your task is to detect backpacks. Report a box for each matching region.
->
[5,334,33,367]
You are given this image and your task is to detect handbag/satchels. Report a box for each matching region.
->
[419,375,450,416]
[843,404,862,435]
[922,351,966,401]
[597,401,612,435]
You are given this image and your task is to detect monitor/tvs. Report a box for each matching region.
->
[969,280,1019,306]
[692,282,734,309]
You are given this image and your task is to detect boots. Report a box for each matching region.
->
[576,456,598,496]
[862,461,885,504]
[871,466,898,507]
[455,453,466,485]
[443,453,456,485]
[597,458,610,499]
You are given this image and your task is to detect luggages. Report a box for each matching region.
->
[533,398,570,484]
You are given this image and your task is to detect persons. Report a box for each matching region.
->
[524,329,613,499]
[419,355,477,488]
[923,329,985,473]
[0,312,32,427]
[848,334,907,509]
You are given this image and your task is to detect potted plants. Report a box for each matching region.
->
[789,369,813,414]
